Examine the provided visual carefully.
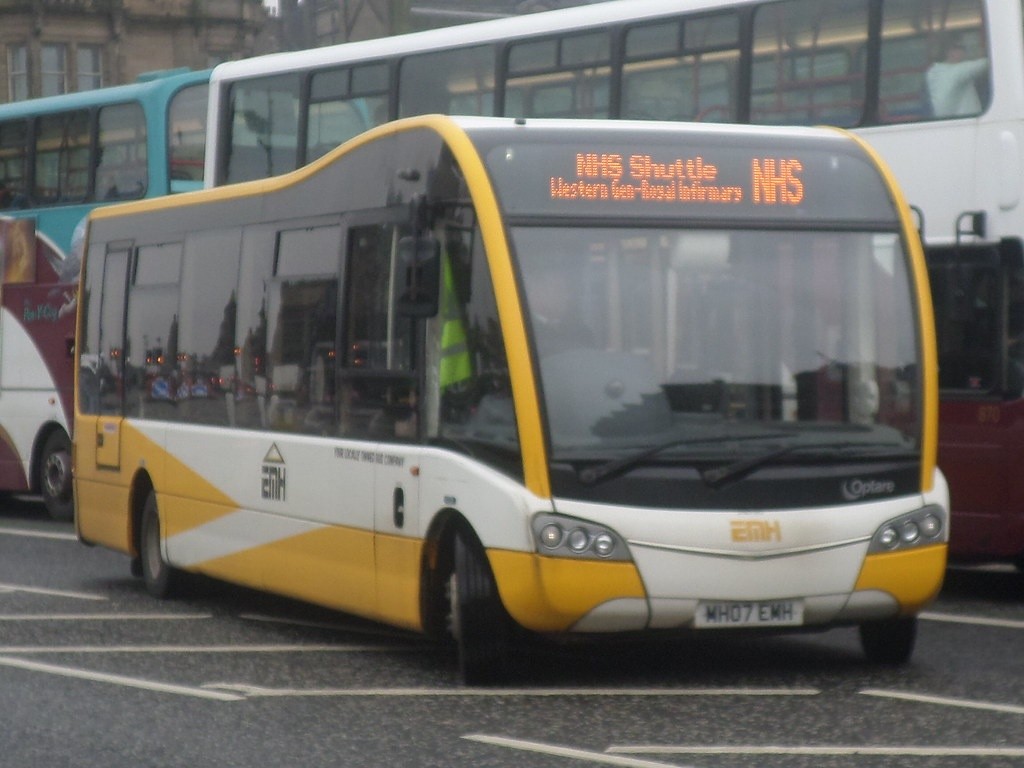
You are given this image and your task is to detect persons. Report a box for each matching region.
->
[926,29,990,118]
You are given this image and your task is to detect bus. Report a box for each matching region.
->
[0,67,218,521]
[213,0,1024,575]
[67,116,953,689]
